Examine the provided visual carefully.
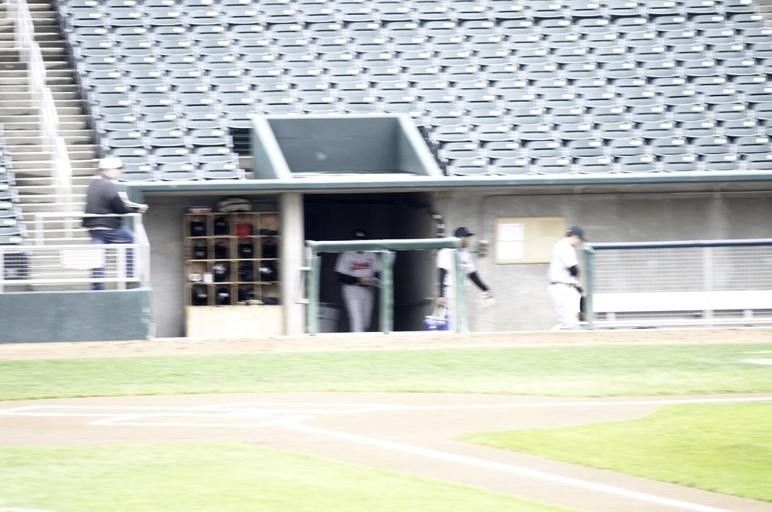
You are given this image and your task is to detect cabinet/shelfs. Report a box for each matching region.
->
[181,212,283,336]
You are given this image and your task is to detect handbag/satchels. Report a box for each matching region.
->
[421,304,450,331]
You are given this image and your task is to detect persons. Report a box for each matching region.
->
[82,157,148,291]
[436,227,495,331]
[547,225,588,329]
[332,230,383,332]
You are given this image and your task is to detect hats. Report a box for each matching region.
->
[565,225,589,241]
[453,226,477,238]
[98,155,125,170]
